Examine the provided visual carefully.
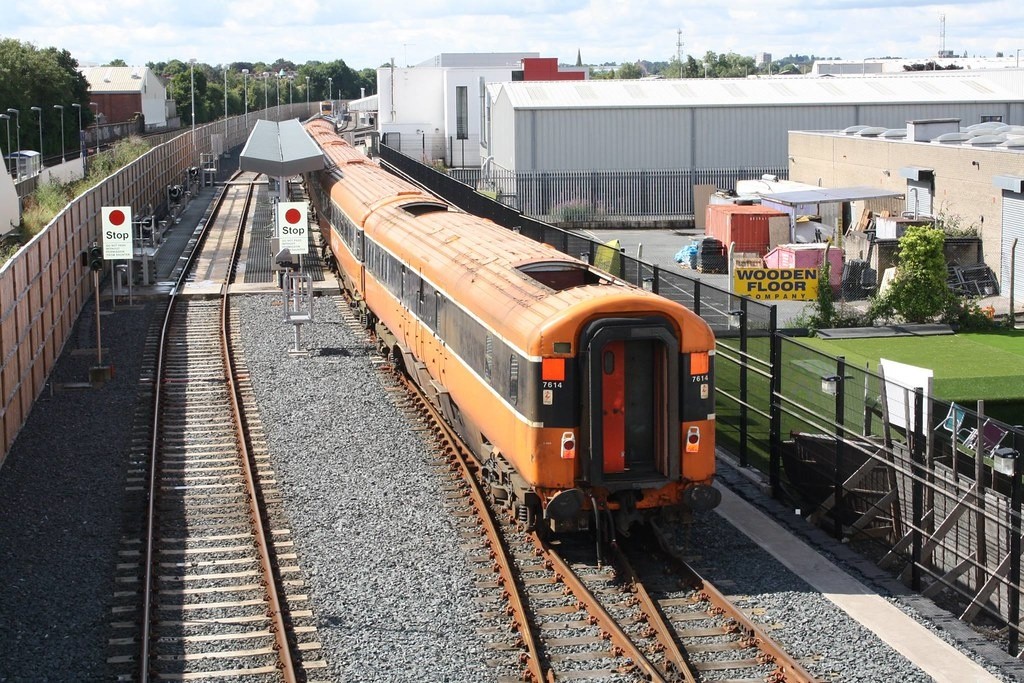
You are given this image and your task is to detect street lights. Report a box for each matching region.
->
[190,58,332,150]
[0,102,100,181]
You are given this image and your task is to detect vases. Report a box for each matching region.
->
[983,286,994,295]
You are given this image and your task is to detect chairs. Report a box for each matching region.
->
[932,401,972,443]
[963,418,1008,458]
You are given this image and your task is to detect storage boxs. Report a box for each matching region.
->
[705,204,789,269]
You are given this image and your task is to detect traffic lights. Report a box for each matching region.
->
[88,246,103,271]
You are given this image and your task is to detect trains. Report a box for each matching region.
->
[304,116,722,539]
[318,99,354,117]
